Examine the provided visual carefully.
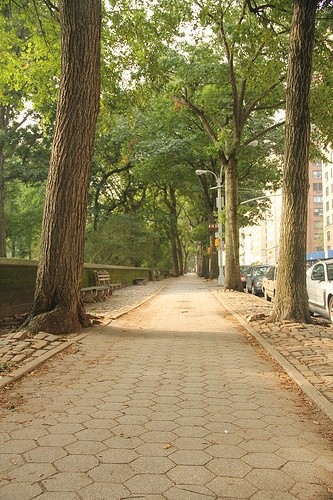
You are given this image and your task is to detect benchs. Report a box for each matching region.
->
[81,286,110,303]
[136,278,148,285]
[93,269,121,296]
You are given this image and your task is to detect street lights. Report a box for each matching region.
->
[195,164,225,286]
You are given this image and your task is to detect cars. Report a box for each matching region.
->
[305,258,333,324]
[221,264,310,303]
